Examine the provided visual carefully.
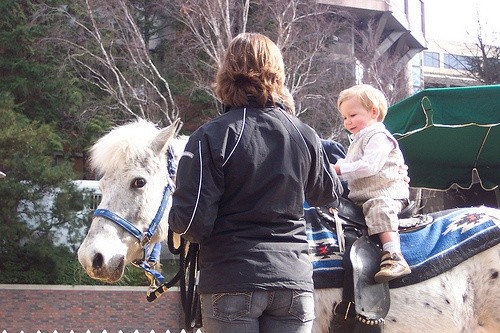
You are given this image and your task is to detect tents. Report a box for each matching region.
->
[344,84,500,192]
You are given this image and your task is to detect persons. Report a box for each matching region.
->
[330,84,411,283]
[168,32,344,333]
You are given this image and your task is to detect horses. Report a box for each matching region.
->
[77,118,500,333]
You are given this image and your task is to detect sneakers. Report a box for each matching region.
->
[374,251,411,283]
